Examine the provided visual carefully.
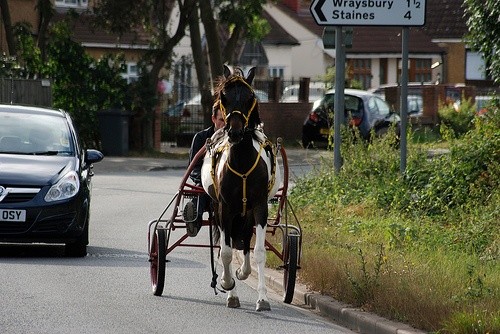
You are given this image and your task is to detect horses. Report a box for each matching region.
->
[201,63,281,312]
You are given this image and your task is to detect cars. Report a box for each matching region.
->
[302,87,400,149]
[180,79,500,137]
[0,103,104,259]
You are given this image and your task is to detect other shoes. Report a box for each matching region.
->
[183,201,201,237]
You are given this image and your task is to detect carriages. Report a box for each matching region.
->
[146,64,304,312]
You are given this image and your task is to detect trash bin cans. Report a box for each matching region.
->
[94,108,134,155]
[409,114,434,145]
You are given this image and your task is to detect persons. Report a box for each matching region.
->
[184,99,226,238]
